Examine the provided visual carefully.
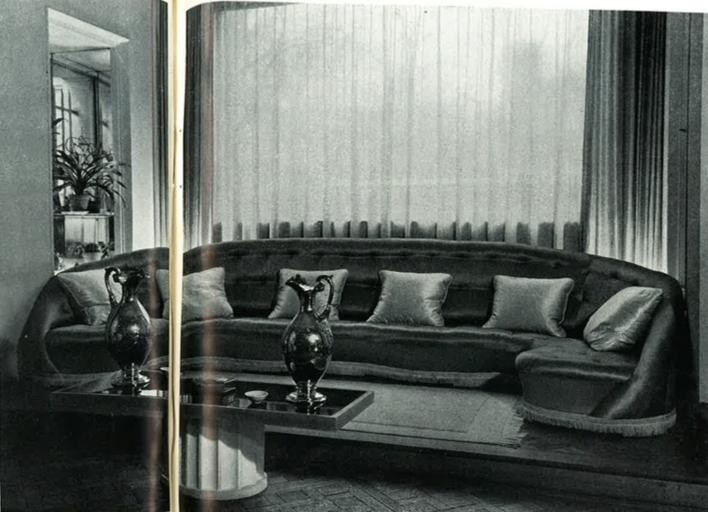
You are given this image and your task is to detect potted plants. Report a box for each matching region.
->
[52,135,128,212]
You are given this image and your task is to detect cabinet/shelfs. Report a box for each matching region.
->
[53,212,110,252]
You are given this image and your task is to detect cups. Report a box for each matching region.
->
[159,366,168,377]
[245,389,270,404]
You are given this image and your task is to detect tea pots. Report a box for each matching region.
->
[104,265,155,388]
[278,275,338,408]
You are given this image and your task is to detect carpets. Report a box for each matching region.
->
[178,366,531,449]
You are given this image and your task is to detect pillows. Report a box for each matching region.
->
[582,286,663,351]
[265,268,350,322]
[480,274,574,339]
[153,266,235,324]
[364,268,454,328]
[55,265,128,328]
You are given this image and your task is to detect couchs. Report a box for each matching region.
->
[16,238,686,441]
